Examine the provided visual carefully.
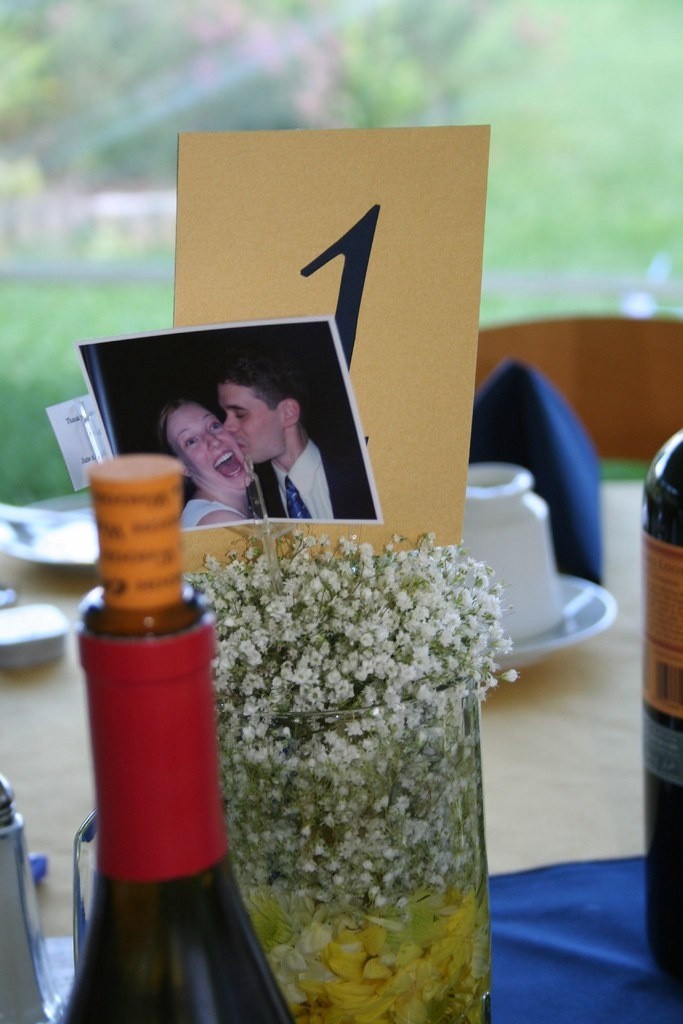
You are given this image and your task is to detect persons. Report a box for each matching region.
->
[157,397,261,530]
[217,351,376,519]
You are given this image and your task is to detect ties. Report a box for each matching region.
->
[284,476,312,519]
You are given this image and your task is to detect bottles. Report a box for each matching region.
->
[639,429,683,979]
[60,453,294,1024]
[0,769,62,1024]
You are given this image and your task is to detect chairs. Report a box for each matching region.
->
[472,317,683,466]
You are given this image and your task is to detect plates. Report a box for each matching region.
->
[473,571,619,671]
[0,489,101,569]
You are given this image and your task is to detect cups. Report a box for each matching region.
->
[462,462,561,641]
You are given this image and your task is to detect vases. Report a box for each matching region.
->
[215,679,490,1024]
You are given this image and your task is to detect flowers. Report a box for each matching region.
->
[186,526,517,1024]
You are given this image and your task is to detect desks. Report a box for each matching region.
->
[0,482,683,1024]
